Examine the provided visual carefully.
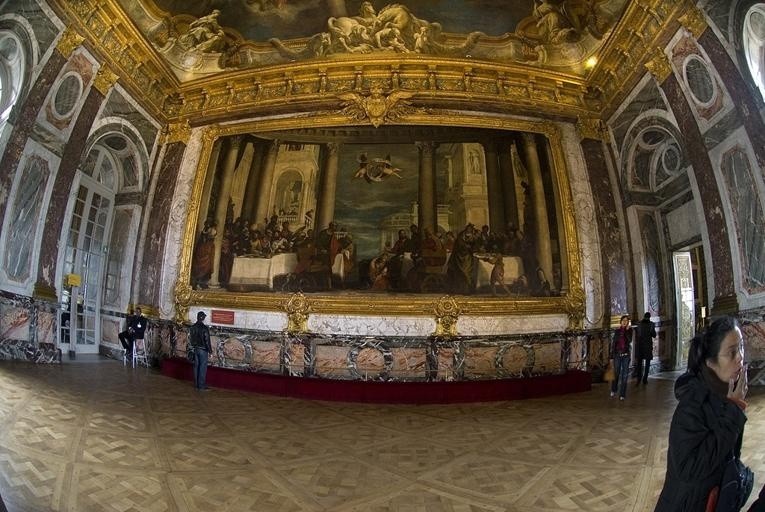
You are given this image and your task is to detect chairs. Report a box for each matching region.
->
[123,319,149,369]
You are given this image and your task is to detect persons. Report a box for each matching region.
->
[119,308,146,356]
[191,312,212,389]
[611,315,634,401]
[636,313,656,385]
[189,9,224,52]
[654,317,748,512]
[375,28,400,51]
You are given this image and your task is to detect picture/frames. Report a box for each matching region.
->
[173,83,586,336]
